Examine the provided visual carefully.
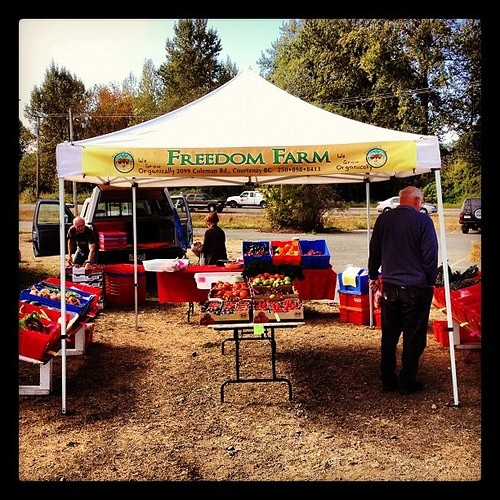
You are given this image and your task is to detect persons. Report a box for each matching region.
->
[66,214,101,269]
[196,210,229,268]
[366,184,440,397]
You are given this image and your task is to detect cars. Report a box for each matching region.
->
[377,196,438,216]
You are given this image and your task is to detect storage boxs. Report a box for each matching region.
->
[16,217,481,395]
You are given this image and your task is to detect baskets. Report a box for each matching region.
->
[433,270,482,346]
[18,220,384,360]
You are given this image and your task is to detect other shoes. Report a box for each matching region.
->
[398,381,426,394]
[383,374,399,393]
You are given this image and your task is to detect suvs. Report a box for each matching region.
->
[458,196,482,235]
[227,190,272,210]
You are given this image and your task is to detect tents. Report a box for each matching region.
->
[53,68,462,414]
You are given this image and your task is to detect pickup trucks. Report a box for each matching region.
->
[185,193,226,214]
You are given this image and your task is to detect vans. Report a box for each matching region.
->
[33,181,195,272]
